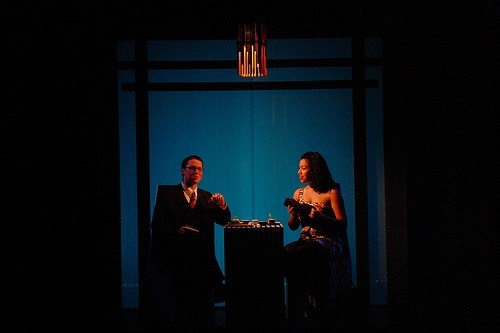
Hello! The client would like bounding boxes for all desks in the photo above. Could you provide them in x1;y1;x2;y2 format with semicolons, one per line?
223;221;285;325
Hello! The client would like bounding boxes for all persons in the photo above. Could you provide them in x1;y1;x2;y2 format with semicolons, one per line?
151;155;231;325
279;149;348;323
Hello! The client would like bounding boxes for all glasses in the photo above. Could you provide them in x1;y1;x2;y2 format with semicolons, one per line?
184;166;204;171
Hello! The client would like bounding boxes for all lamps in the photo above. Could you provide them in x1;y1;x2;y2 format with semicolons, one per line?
235;13;268;78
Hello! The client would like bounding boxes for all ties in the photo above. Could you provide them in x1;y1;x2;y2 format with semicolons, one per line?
189;191;197;209
